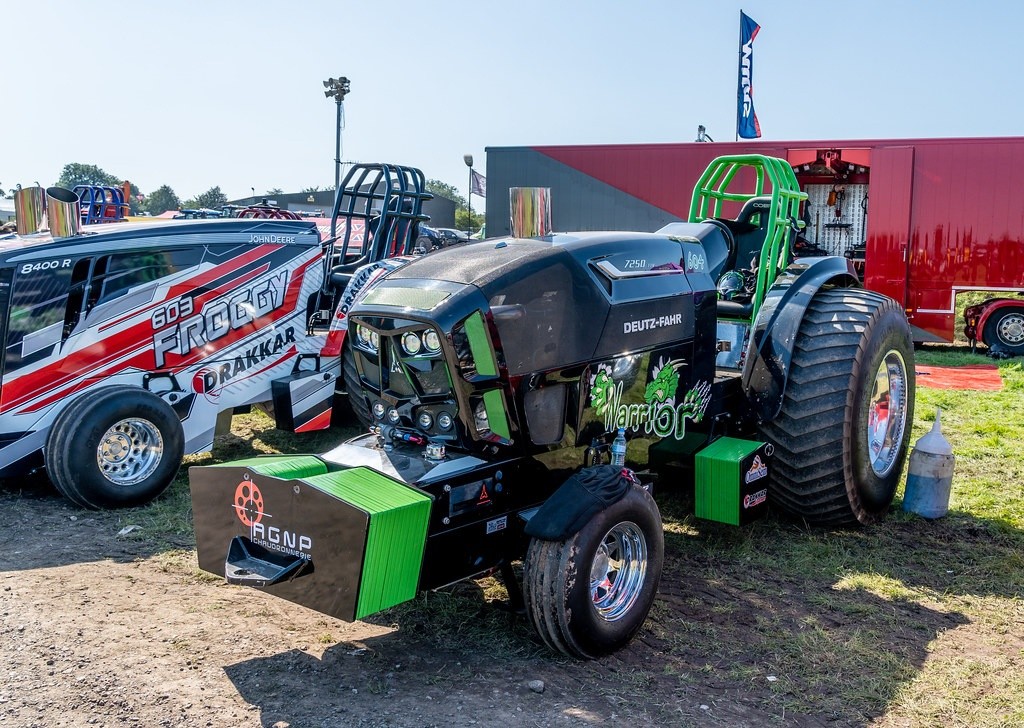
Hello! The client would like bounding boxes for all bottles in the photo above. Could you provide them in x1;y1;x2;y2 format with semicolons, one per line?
903;409;955;518
610;428;627;467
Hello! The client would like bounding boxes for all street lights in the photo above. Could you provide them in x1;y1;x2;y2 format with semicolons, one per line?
324;75;351;217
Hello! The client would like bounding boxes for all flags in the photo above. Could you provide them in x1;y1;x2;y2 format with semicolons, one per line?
471;168;486;197
738;9;761;139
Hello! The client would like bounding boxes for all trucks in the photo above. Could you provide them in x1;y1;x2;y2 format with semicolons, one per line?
484;135;1024;360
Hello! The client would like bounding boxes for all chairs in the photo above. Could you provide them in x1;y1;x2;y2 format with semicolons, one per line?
732;196;781;272
332;198;414;287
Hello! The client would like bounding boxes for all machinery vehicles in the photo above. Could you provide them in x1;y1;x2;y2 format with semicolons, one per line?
0;162;458;512
189;154;915;665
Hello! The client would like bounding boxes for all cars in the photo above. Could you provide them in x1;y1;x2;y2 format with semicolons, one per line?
435;228;469;243
